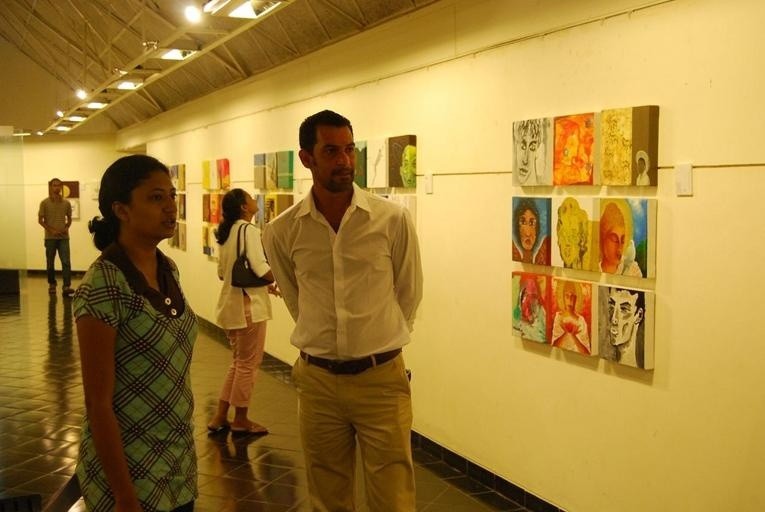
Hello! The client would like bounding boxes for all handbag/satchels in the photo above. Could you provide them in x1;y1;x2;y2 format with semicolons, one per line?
231;254;275;288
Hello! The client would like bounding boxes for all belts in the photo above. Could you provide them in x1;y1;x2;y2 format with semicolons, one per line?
299;347;403;376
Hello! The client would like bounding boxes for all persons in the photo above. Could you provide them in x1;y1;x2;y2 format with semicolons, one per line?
205;185;275;436
599;199;643;279
513;197;549;265
37;176;76;296
606;287;645;369
513;118;544;185
72;151;203;512
551;280;591;356
554;196;589;269
43;293;78;425
207;427;267;511
259;107;427;512
396;145;416;187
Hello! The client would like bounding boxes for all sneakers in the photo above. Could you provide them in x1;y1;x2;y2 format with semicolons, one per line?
49;280;57;294
62;286;75;295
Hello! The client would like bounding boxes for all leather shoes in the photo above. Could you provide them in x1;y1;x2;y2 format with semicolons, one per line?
230;422;270;434
207;419;231;432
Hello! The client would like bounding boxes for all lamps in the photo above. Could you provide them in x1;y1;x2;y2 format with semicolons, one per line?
30;0;296;137
